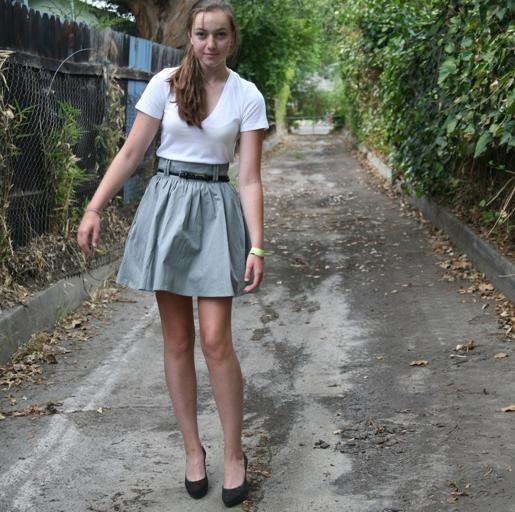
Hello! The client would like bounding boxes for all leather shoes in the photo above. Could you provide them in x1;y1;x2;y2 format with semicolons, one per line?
222;453;248;507
185;445;207;498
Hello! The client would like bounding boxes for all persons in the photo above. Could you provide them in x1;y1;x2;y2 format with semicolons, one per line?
77;0;269;507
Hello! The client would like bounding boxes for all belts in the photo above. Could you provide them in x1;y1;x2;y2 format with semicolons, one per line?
157;170;229;181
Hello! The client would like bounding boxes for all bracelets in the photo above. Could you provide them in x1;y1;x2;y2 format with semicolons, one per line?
249;247;266;258
84;208;102;216
